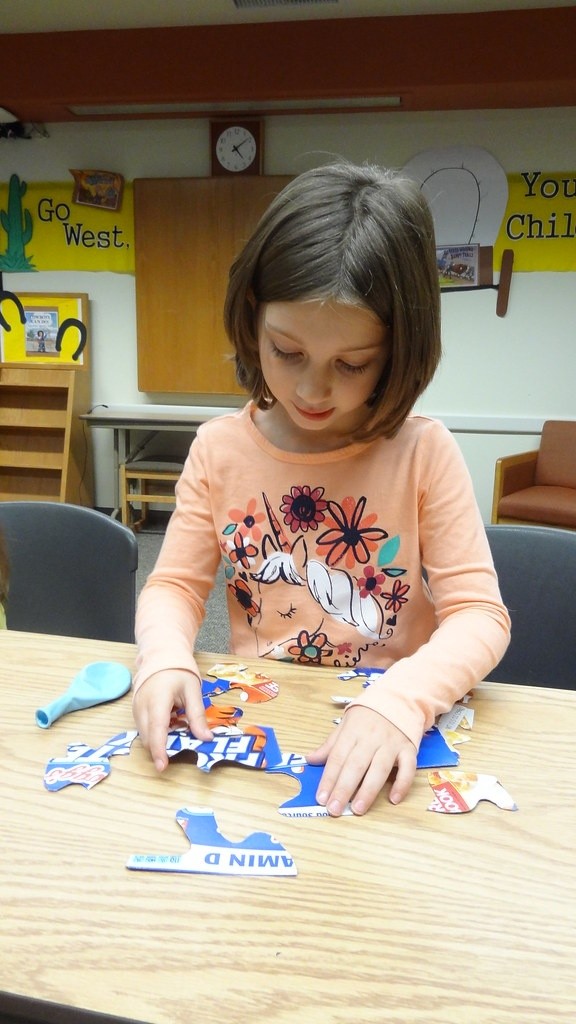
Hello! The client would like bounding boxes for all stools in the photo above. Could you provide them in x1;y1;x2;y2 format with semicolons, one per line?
120;456;189;526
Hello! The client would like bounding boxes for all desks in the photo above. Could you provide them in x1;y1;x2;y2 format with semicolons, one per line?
0;631;576;1024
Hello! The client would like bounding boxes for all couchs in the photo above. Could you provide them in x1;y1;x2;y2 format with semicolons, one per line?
491;420;576;531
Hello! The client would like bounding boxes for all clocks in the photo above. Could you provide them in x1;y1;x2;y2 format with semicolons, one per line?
209;120;264;176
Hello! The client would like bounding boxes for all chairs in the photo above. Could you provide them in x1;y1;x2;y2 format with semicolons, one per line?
483;525;576;692
0;500;139;643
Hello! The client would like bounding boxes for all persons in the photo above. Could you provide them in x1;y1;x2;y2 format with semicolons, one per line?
132;151;513;818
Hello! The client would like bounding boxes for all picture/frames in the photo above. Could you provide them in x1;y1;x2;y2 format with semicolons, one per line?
0;292;90;371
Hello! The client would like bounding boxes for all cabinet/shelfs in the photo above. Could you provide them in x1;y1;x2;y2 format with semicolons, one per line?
0;370;94;508
